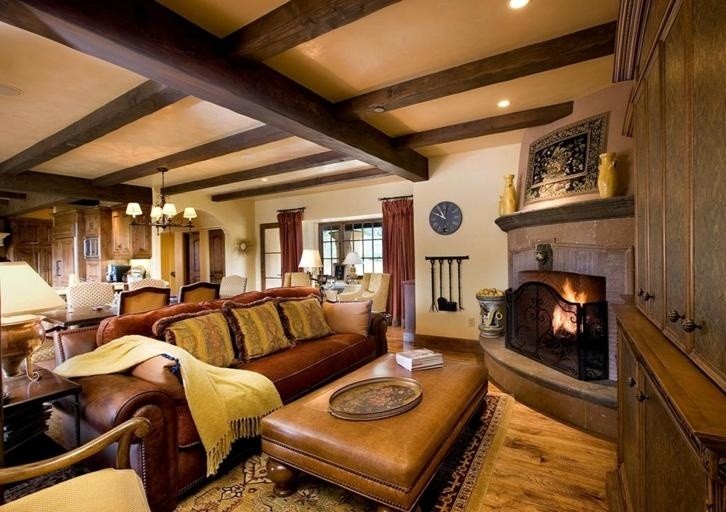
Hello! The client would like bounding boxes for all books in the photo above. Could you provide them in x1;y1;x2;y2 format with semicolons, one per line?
395;347;443;372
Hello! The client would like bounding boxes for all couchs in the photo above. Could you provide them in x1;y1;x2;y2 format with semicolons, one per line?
22;286;390;512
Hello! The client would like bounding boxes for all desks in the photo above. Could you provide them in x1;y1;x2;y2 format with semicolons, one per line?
0;363;83;469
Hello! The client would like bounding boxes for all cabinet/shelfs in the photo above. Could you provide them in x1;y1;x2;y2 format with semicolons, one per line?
111;205;135;259
52;237;81;284
628;55;663;331
615;332;708;511
660;0;726;385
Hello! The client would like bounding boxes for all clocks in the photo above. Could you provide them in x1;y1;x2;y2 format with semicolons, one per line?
429;202;463;234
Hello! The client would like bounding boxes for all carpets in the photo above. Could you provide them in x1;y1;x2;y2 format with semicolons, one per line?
157;366;513;511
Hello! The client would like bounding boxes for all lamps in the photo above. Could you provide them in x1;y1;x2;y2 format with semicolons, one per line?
297;249;323;286
125;166;198;235
342;252;363;279
0;262;67;382
